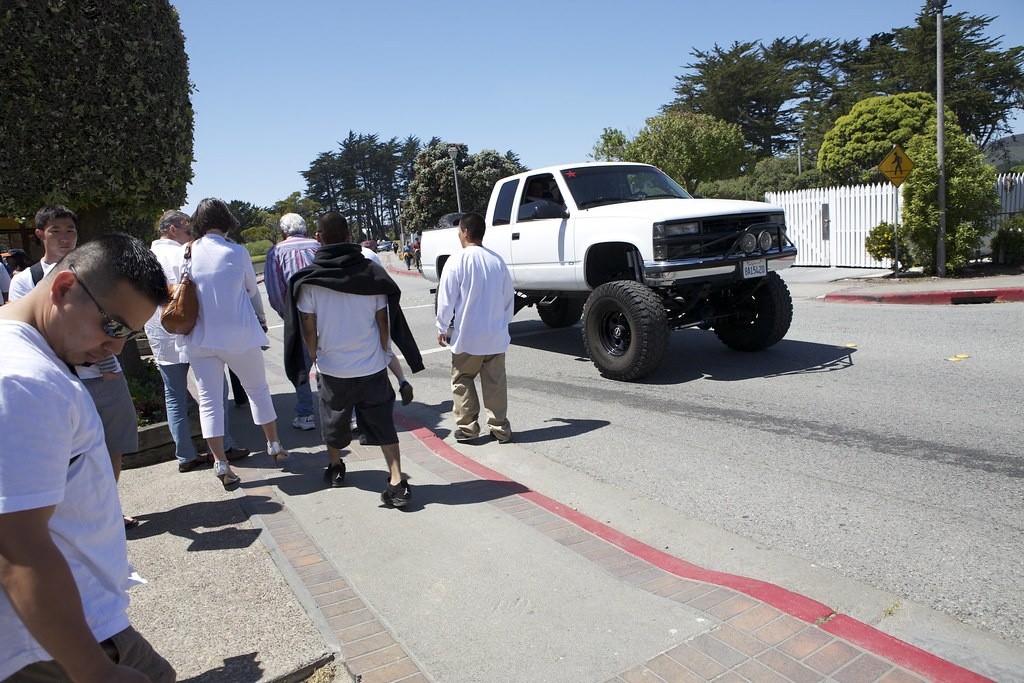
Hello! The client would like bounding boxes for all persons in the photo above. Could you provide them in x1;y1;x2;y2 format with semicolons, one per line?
435;212;514;444
413;238;420;269
0;233;170;683
264;213;322;430
144;209;251;473
284;211;414;507
393;242;398;254
8;206;139;529
402;240;413;270
177;197;290;490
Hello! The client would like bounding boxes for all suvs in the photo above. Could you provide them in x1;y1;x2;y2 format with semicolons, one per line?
360;240;377;253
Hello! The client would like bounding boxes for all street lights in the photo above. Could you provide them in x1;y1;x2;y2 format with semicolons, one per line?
448;147;461;212
395;198;406;247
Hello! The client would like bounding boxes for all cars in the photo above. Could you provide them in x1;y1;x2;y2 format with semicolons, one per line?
376;241;392;252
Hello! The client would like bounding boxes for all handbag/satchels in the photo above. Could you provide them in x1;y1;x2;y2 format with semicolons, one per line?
162;272;200;335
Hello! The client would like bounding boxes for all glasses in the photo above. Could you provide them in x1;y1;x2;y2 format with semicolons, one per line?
172;225;192;236
69;263;142;342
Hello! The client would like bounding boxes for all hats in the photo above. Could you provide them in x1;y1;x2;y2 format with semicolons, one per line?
1;249;20;258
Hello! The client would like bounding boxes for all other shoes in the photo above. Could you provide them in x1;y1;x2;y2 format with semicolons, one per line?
453;429;477;441
400;380;414;406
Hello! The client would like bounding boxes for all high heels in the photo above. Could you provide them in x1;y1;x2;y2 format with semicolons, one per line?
214;461;239;490
267;440;290;469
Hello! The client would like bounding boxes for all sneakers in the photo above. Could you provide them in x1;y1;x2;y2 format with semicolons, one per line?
178;454;207;473
350;414;359;433
380;477;412;507
324;459;346;487
208;447;251;464
292;414;316;430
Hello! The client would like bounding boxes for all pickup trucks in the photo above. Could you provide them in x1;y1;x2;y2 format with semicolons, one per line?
421;161;798;381
